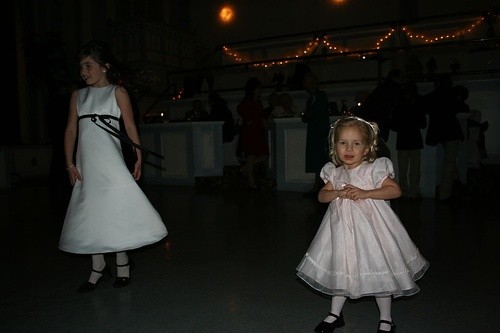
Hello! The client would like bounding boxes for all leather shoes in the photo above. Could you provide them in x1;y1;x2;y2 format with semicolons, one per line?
77;264;111;293
315;310;345;333
113;256;133;287
377;315;396;333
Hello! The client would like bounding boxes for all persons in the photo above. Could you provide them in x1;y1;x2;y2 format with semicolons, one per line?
56;42;170;294
296;116;431;333
185;70;488;203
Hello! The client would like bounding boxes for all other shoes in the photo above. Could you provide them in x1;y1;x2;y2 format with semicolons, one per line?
303;187;321;197
